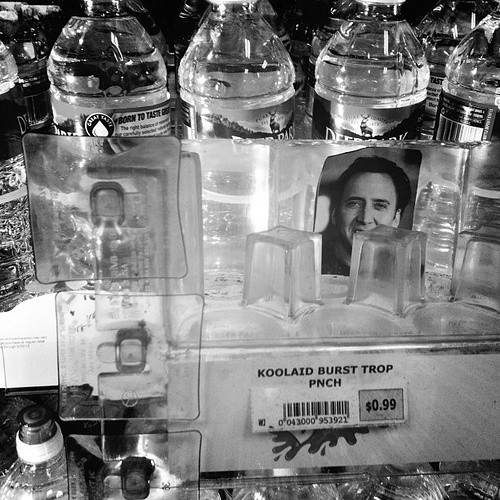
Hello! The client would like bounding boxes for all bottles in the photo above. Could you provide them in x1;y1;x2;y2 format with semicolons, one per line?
2;388;499;500
0;0;500;318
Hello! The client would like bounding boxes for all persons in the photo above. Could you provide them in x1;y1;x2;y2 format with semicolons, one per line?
321;155;414;277
